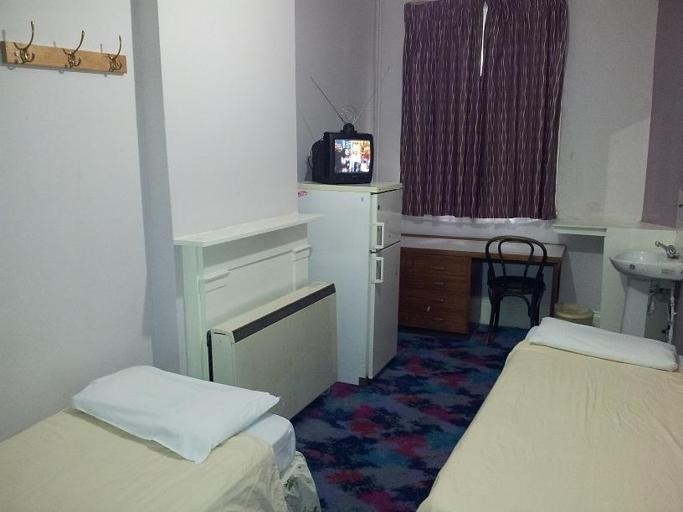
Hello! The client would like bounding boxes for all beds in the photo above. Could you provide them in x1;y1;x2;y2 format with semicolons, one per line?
0;408;320;512
417;324;683;512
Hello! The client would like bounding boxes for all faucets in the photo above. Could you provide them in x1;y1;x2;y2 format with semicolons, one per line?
655;241;680;258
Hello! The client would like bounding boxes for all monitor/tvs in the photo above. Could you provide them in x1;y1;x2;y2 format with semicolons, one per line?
311;132;374;184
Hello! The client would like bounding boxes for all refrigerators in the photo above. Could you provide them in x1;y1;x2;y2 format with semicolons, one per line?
298;181;401;386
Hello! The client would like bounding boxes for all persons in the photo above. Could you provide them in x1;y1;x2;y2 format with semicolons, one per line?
340;148;351;173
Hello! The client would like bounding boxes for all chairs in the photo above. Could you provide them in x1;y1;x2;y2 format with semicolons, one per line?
483;235;548;348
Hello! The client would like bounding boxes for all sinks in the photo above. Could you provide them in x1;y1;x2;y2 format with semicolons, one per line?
609;249;683;281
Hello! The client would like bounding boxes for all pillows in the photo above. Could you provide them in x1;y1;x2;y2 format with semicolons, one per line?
528;316;680;373
69;366;285;466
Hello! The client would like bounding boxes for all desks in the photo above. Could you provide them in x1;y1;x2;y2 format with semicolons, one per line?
399;233;567;343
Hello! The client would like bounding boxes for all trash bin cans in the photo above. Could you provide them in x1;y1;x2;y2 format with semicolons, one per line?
555;303;593;326
